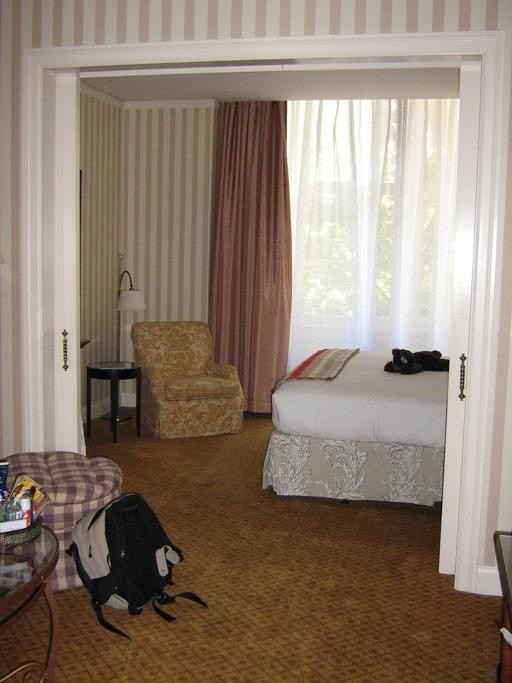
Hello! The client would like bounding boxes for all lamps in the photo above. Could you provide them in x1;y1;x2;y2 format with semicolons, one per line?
103;249;147;422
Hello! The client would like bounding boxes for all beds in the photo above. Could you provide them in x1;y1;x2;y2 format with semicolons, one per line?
261;347;449;507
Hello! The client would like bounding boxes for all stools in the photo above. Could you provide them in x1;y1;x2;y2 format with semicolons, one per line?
1;450;122;593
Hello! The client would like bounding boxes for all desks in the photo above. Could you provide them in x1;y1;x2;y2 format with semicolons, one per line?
85;360;142;445
0;523;63;683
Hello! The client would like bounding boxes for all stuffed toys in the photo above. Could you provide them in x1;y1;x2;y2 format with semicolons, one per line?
384;347;449;374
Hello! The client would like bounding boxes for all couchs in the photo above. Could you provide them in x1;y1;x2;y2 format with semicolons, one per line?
131;318;248;440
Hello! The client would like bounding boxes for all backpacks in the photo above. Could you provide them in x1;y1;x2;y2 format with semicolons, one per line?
65;493;208;641
